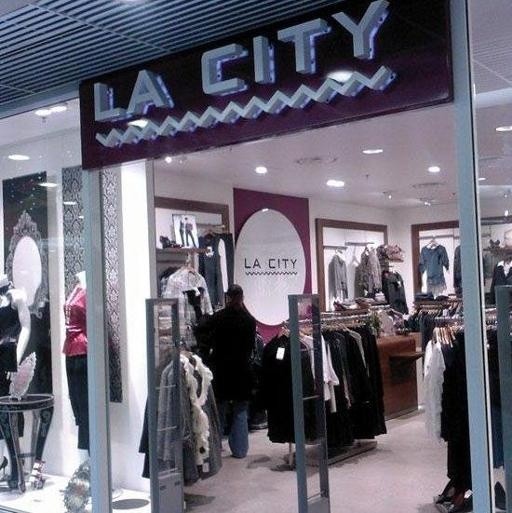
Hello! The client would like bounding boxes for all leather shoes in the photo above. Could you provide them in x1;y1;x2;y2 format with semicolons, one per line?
436;476;457;504
449;494;472;512
495;481;506;509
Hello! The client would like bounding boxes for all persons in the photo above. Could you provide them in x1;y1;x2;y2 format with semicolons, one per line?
62;270;90;457
194;284;255;458
489;245;512;305
0;273;34;483
179;215;185;247
184;218;196;248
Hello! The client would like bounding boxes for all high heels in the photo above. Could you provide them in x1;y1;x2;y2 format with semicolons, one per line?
0;455;9;477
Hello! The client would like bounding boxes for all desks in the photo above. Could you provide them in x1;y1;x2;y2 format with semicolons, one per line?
0;393;54;485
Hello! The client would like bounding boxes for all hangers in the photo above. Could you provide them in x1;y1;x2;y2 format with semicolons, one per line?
417;232;445;251
264;310;371;353
414;301;512;357
157;262;206;286
329;242;374;266
